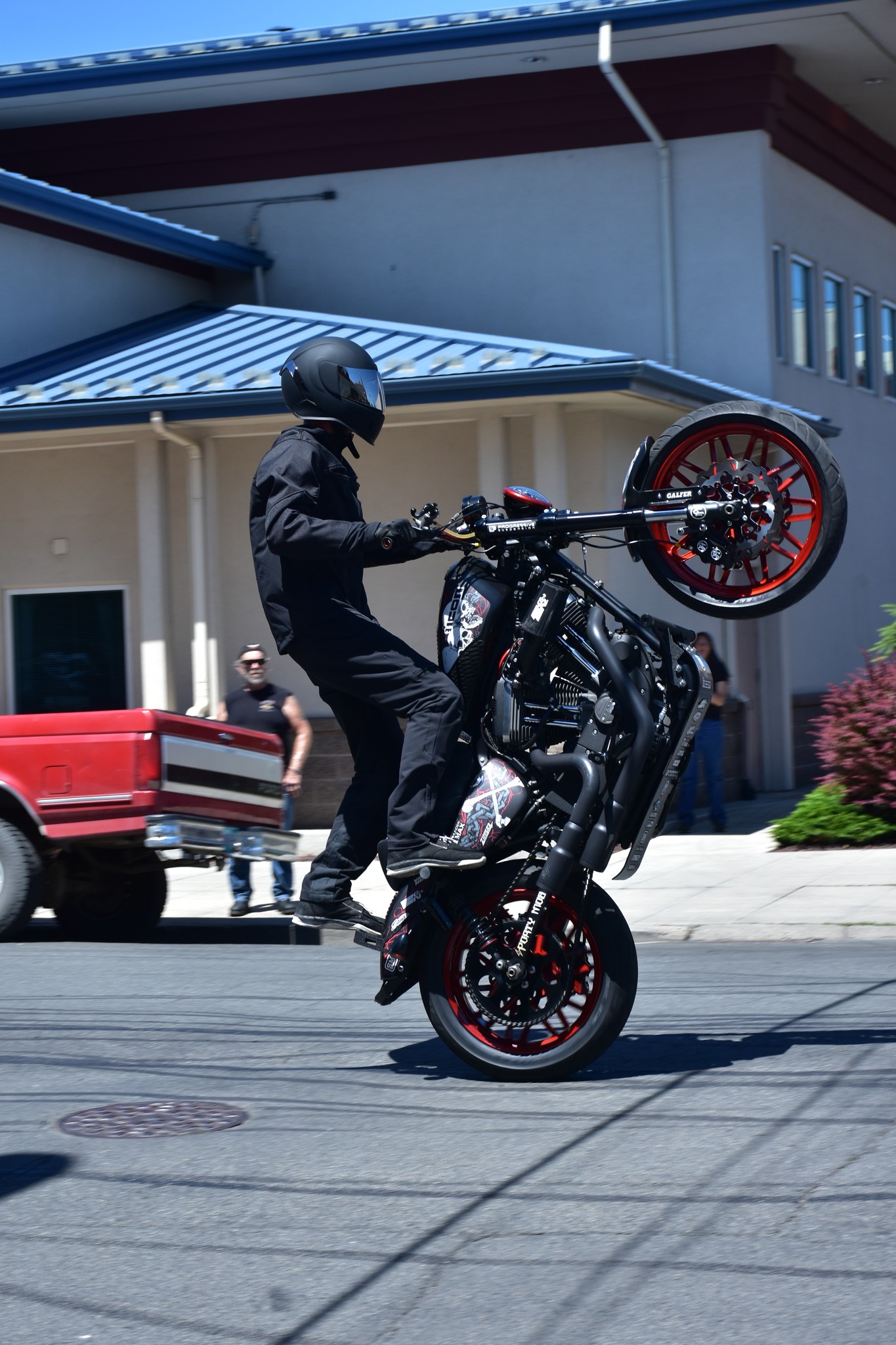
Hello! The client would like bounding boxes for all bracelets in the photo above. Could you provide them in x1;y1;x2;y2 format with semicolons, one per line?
287;767;304;776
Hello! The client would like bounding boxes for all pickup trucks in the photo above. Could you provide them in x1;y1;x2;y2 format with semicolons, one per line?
0;708;299;939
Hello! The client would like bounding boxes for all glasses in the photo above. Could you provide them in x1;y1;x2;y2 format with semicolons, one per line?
242;658;265;666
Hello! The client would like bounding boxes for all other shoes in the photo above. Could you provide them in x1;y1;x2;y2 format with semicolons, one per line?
277;898;297;915
679;825;693;833
231;901;248;916
714;822;728;832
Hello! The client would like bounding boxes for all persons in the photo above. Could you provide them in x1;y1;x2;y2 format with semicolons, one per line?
672;631;728;835
251;343;487;928
214;643;314;917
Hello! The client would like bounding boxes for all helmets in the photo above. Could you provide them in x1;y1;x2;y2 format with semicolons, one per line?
278;337;384;446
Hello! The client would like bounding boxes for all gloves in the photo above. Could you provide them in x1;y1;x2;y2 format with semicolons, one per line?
374;518;417;547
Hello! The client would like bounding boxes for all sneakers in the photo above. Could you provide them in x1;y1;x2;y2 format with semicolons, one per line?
291;899;384;937
385;838;486;878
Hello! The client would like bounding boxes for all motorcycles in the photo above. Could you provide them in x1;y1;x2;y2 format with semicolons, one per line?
370;399;850;1078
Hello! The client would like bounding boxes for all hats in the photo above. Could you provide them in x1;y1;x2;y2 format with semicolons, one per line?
238;643;266;659
